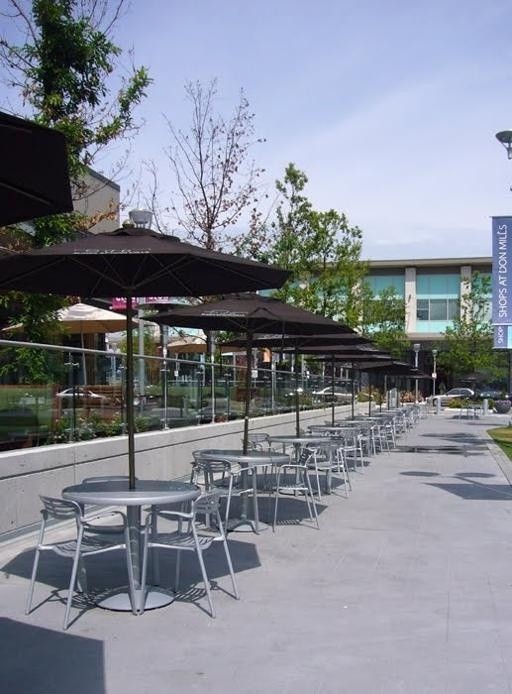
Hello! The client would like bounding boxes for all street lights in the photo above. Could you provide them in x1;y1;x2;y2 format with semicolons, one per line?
127;207;157;396
494;129;512;191
431;348;438;397
413;342;420;406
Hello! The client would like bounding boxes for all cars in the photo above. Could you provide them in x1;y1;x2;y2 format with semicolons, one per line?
51;385;111;405
138;405;200;420
282;386;311;398
473;387;512;406
181;388;281;421
123;393;161;412
426;387;476;405
311;385;358;402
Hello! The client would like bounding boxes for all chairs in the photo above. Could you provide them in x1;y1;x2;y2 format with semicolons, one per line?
457;399;490;422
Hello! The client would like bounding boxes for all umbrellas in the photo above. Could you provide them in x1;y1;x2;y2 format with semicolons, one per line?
0;294;138;417
0;217;296;490
0;109;75;228
